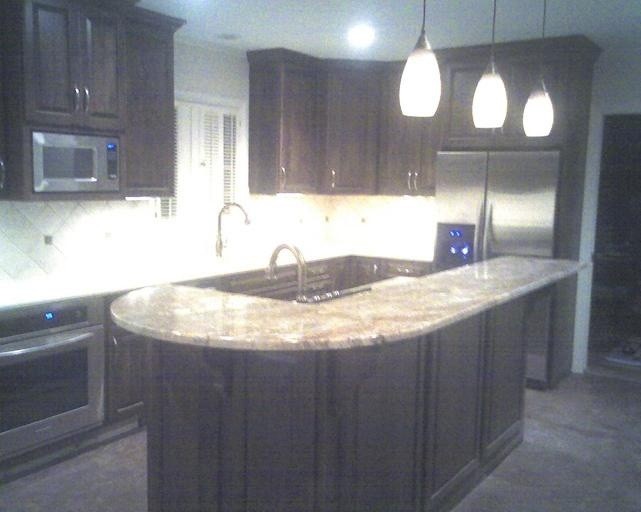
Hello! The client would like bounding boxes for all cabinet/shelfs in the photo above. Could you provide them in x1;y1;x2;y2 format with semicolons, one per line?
352;255;435;288
322;62;379;195
250;52;320;193
0;2;123;128
379;62;443;196
228;258;350;298
445;33;604;148
105;278;221;422
120;7;176;198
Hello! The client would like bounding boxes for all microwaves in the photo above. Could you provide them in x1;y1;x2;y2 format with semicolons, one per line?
30;128;120;194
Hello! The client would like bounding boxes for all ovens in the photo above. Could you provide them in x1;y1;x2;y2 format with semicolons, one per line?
0;294;106;460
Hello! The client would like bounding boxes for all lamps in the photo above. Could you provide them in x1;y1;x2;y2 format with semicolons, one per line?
398;2;444;119
522;0;556;139
471;0;509;129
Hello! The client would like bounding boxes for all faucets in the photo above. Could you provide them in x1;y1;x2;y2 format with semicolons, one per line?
216;198;252;264
264;241;312;303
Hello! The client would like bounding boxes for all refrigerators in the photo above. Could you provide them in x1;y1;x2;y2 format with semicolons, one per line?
433;149;559;388
